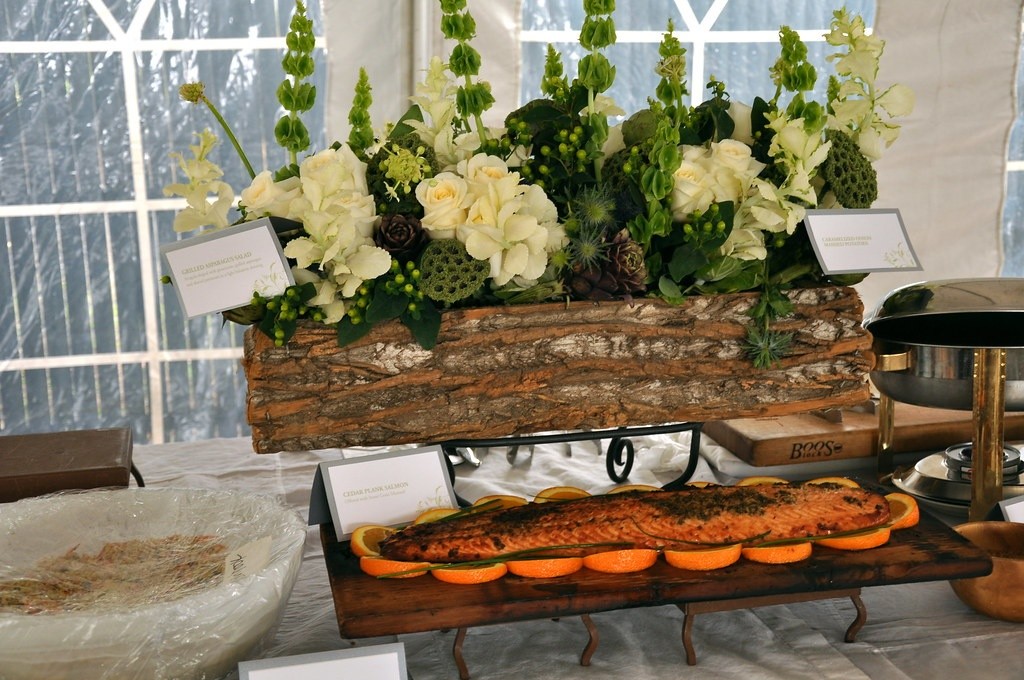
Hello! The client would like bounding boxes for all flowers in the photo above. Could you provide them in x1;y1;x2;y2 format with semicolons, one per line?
165;0;915;348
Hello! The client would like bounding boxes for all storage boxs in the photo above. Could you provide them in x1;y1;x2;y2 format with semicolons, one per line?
697;401;1022;465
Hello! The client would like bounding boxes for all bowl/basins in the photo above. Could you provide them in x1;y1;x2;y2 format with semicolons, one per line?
947;521;1024;624
0;489;308;680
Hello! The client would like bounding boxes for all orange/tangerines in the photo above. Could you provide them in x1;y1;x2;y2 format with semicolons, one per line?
350;475;918;583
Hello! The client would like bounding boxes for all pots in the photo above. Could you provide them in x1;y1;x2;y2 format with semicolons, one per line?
890;441;1024;518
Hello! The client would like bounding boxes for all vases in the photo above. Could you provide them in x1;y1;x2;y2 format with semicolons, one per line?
245;270;880;452
